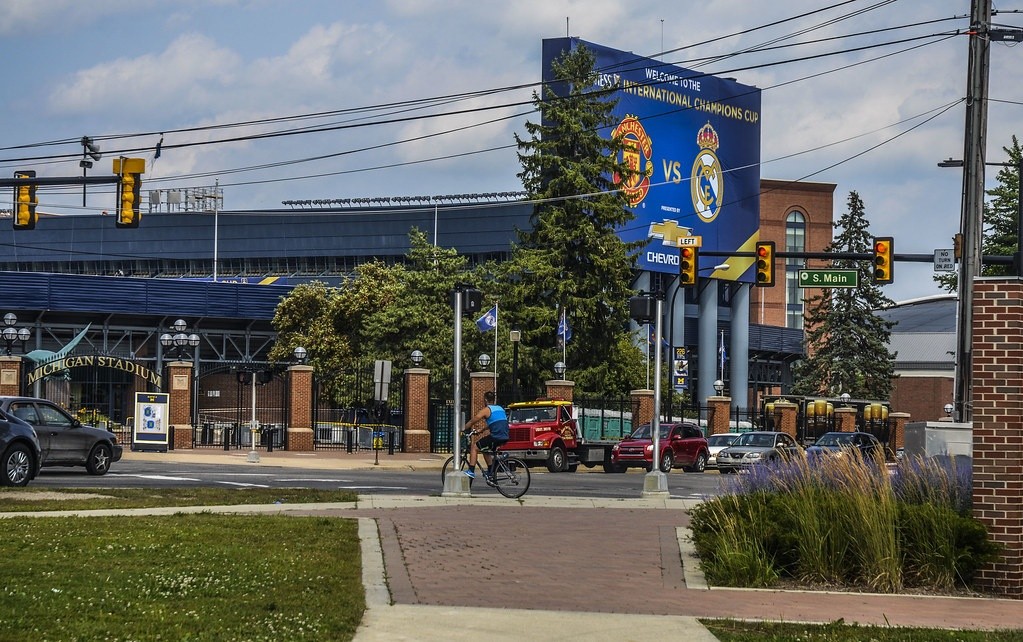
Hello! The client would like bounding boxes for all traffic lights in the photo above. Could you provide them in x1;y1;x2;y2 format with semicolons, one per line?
258;370;274;383
875;240;890;281
757;245;772;284
236;370;252;384
680;247;694;284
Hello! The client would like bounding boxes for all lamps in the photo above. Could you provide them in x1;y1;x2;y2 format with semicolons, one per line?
554;362;566;380
158;319;200;361
478;353;491;372
282;189;532;208
712;379;724;396
943;403;954;417
293;346;307;365
839;393;851;408
410;350;424;368
0;312;32;355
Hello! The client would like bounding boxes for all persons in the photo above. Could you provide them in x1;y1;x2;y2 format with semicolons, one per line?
463;392;510;479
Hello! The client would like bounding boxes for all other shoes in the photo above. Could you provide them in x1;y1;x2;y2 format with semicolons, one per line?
463;469;475;479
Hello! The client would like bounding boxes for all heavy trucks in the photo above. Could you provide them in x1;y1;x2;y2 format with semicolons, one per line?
498;399;634;476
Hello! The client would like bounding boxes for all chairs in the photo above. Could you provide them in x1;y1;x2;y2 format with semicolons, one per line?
15;408;37;425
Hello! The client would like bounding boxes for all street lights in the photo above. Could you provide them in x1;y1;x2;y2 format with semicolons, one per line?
668;264;729;423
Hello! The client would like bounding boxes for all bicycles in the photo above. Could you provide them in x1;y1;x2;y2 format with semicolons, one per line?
442;429;530;498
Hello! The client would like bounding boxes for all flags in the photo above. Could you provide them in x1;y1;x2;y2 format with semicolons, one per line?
475;306;496;332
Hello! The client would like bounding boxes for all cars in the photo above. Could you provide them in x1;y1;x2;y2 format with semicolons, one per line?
803;431;883;465
612;421;710;472
716;431;800;475
703;433;744;470
0;395;122;476
0;407;40;487
